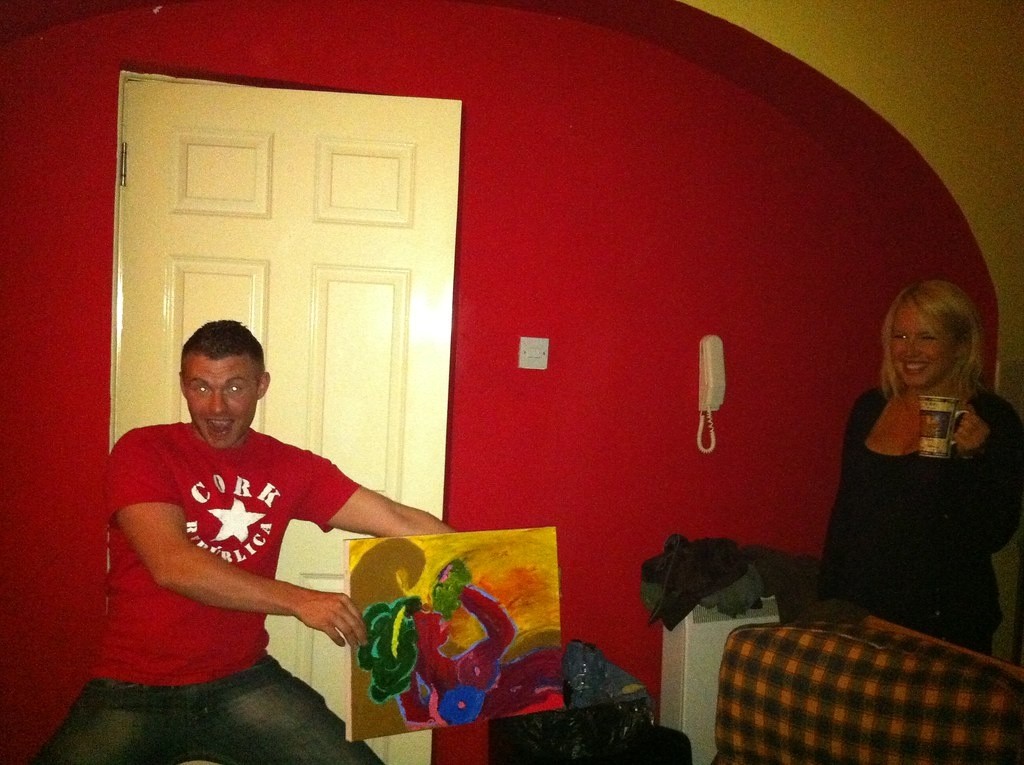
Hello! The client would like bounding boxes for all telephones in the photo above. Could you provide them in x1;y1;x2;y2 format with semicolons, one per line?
698;334;726;412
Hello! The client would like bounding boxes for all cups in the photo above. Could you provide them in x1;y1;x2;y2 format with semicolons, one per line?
917;395;969;459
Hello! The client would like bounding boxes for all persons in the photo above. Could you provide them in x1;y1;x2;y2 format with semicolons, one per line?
32;320;458;765
819;278;1024;656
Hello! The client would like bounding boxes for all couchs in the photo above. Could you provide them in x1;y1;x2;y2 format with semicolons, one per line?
709;607;1024;765
